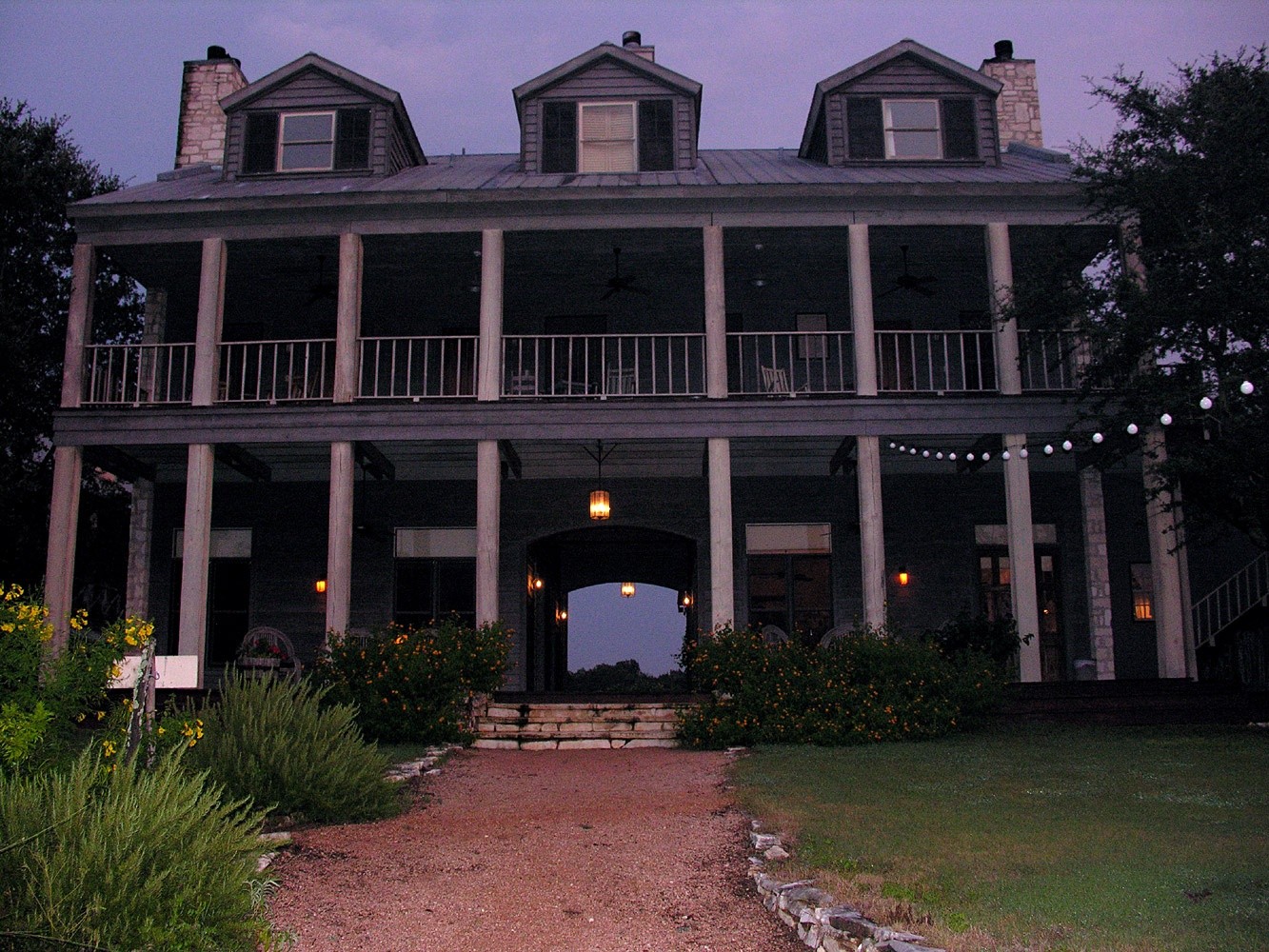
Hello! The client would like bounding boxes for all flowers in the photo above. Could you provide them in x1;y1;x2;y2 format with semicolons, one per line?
238;640;282;658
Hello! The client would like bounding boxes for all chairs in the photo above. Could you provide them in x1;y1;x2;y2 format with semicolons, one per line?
820;622;863;651
760;365;811;400
762;625;788;645
285;365;320;398
244;625;302;682
604;363;640;400
318;631;373;652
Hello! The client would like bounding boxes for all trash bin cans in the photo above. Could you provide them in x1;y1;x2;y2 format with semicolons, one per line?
1073;656;1097;681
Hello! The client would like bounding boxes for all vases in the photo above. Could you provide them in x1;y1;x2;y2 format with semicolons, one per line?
240;658;280;689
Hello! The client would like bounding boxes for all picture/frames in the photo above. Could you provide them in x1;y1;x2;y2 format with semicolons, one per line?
795;312;829;361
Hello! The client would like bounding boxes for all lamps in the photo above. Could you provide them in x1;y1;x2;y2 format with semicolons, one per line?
620;581;637;600
585;441;617;521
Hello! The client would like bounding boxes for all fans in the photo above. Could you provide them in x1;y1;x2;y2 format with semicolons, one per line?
284;254;338;300
581;248;653;302
875;245;938;298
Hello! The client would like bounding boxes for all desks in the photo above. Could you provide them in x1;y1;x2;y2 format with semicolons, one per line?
510;369;541;398
188;381;229;404
548;381;597;398
